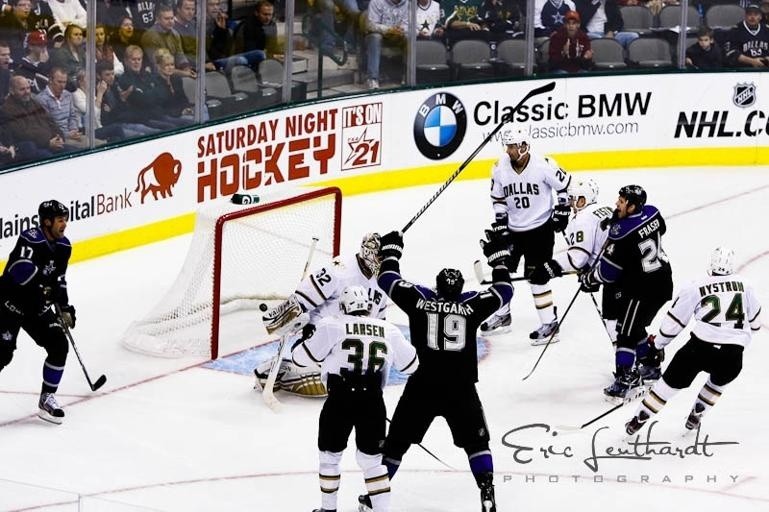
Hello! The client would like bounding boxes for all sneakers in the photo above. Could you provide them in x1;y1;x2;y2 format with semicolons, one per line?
480;313;511;331
603;372;642;398
364;78;379;90
38;392;65;417
358;494;372;509
481;486;496;512
684;409;702;430
528;320;560;339
624;415;647;435
633;366;662;380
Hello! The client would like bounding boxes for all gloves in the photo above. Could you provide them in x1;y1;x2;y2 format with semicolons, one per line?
528;260;563;285
645;343;665;367
380;230;405;260
479;229;514;268
577;268;601;293
61;305;76;329
302;323;317;340
553;204;571;233
491;213;508;232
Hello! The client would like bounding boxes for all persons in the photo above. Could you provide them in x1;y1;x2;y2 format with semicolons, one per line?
2;1;767;167
527;175;621;353
577;182;673;396
619;246;767;435
255;233;388;399
0;199;78;416
358;226;514;512
290;285;420;512
479;131;577;341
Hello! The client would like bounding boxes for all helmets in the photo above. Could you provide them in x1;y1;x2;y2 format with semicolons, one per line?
706;243;737;276
338;284;369;314
566;176;600;210
435;268;465;295
618;185;647;207
358;232;383;279
38;199;69;223
502;125;530;146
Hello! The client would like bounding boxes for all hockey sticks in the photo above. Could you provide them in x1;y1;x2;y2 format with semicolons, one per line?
522;237;608;381
262;236;320;414
473;260;578;285
387;418;469;472
555;387;652;431
54;300;107;391
401;82;556;234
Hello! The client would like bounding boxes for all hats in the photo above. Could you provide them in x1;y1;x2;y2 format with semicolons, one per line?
564;11;581;21
26;29;48;46
745;2;761;12
